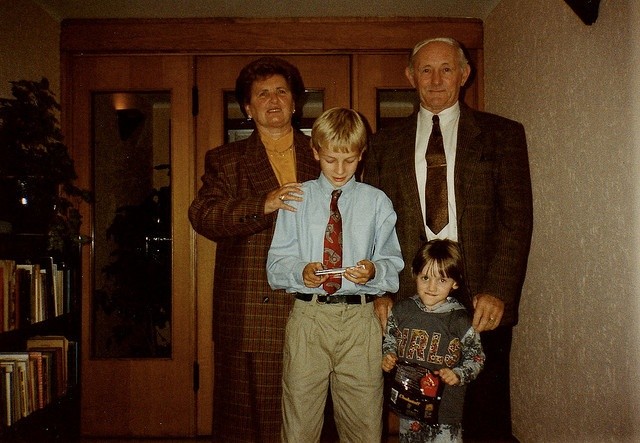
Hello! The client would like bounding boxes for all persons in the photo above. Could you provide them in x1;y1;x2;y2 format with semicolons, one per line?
361;36;535;443
380;238;487;443
265;106;406;443
187;53;322;442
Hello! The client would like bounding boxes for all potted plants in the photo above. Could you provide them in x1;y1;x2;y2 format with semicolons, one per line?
0;75;98;261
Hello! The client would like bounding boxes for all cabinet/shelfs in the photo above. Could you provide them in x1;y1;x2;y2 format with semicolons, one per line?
351;48;481;177
0;260;81;434
63;49;195;439
196;52;351;438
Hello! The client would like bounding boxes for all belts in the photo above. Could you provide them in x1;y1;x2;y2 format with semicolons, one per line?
296;293;373;305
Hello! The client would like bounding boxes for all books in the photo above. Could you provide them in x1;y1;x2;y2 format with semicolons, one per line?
0;335;78;427
0;255;72;333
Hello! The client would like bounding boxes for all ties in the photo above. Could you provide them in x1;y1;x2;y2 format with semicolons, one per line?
322;190;343;294
425;115;450;235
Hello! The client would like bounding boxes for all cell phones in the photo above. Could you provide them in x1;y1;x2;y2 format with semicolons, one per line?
315;265;366;278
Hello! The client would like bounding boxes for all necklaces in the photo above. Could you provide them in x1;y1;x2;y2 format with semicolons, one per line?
264;143;295;157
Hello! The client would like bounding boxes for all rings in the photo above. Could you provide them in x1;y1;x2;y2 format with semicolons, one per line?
278;193;287;200
488;317;495;325
